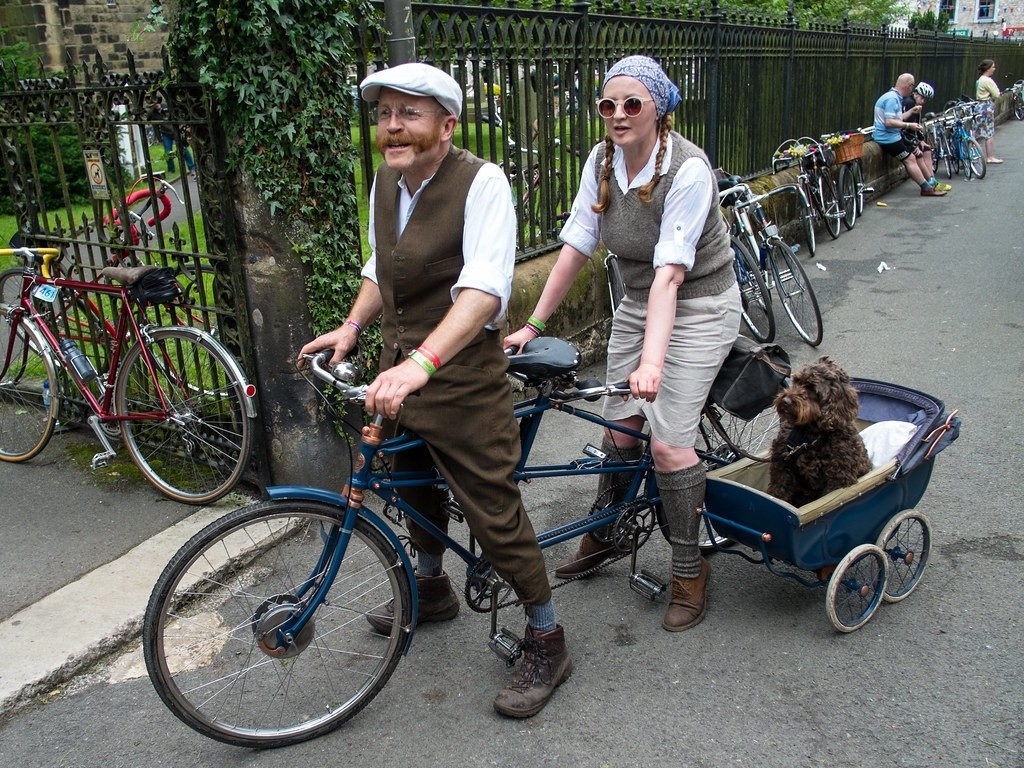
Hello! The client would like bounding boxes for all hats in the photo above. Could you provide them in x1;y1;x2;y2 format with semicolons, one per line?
360;63;463;125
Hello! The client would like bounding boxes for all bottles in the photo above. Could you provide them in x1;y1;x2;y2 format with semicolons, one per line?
59;336;96;382
42;381;60;425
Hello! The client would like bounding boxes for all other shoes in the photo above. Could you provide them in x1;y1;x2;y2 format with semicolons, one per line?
988;156;1004;164
921;187;947;196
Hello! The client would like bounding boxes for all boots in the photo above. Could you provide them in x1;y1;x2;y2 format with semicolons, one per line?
367;569;460;635
495;619;569;718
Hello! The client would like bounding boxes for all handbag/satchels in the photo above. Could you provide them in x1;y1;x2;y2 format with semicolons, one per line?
709;334;792;424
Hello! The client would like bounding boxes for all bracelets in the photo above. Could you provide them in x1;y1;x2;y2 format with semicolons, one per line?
408;349;437;376
525;316;546;336
905;124;910;129
418;346;441;369
345;320;361;332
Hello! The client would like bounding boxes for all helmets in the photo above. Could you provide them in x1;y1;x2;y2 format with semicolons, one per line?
915;81;934;100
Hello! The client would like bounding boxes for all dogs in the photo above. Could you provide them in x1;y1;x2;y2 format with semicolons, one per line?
766;355;872;503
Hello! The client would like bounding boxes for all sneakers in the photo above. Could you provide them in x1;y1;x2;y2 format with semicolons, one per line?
663;557;710;632
555;533;629;577
935;182;952;191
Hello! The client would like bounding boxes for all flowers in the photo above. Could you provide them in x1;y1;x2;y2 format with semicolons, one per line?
819;130;855;149
772;141;817;161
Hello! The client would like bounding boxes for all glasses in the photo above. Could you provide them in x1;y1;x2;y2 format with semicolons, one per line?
369;105;446;123
595;97;654;118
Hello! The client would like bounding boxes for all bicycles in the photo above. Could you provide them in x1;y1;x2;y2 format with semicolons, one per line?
0;177;238;397
716;176;825;348
1009;79;1024;121
772;134;875;257
921;96;987;182
0;247;262;507
141;320;966;749
482;103;591;250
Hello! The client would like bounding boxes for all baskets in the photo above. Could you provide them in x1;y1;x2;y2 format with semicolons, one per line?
832;134;865;164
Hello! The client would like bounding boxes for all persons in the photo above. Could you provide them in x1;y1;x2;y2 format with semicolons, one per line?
973;59;1004;163
574;71;598;99
553;71;559;118
296;64;572;720
503;54;743;631
149;103;195;176
901;82;952;191
872;73;947;196
